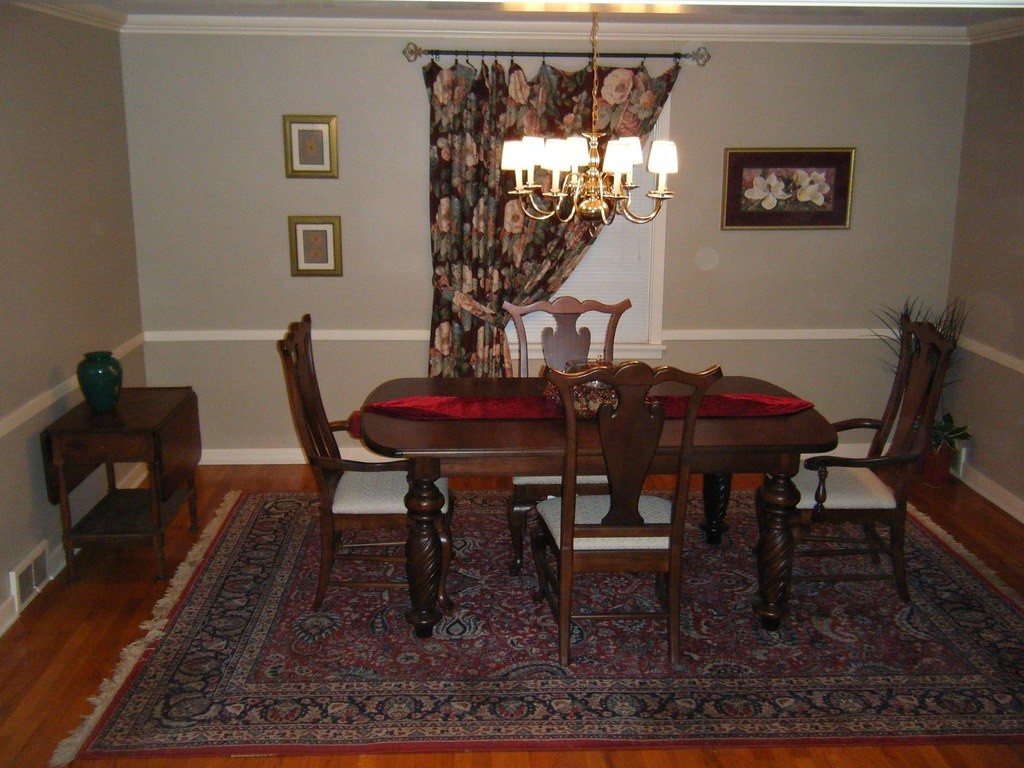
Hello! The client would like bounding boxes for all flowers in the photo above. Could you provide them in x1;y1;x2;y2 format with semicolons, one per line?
544;355;620;415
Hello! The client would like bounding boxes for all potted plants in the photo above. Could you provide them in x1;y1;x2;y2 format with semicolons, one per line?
866;296;972;488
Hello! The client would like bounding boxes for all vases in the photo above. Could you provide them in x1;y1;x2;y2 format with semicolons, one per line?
77;350;121;413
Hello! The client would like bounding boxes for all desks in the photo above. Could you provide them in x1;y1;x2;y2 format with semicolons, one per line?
39;385;203;583
361;376;839;637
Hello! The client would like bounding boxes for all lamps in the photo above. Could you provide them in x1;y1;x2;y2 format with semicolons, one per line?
500;12;678;237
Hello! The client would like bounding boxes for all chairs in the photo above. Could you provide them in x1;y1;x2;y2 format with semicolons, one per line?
750;312;958;601
502;297;633;577
279;314;456;614
530;362;724;665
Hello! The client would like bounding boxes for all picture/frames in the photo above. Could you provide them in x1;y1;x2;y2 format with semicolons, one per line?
721;147;855;230
289;216;342;277
283;114;339;179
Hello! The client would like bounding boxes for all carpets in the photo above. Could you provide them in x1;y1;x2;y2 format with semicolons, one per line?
48;489;1024;765
